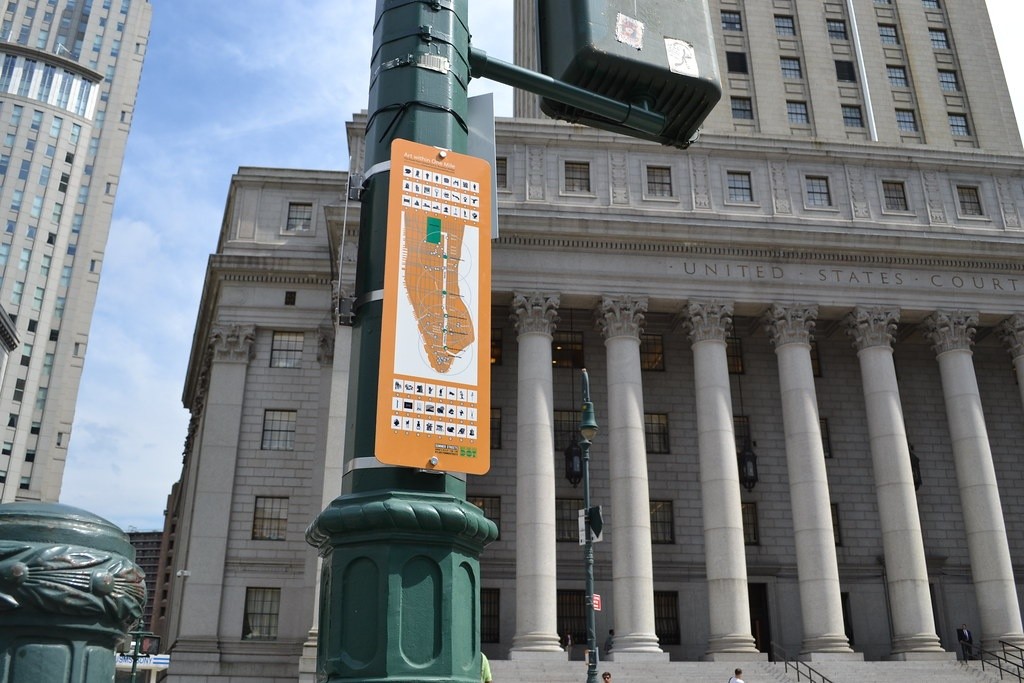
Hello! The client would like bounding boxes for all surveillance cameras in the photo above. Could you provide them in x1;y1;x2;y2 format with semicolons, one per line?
177;571;182;577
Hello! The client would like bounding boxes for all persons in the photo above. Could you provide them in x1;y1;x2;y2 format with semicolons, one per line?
958;624;973;661
480;652;492;683
604;629;615;655
602;672;612;683
728;668;745;683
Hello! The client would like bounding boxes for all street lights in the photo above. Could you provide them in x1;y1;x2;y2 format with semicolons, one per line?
568;367;604;683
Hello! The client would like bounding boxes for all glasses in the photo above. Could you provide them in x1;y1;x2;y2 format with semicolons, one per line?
604;677;610;680
